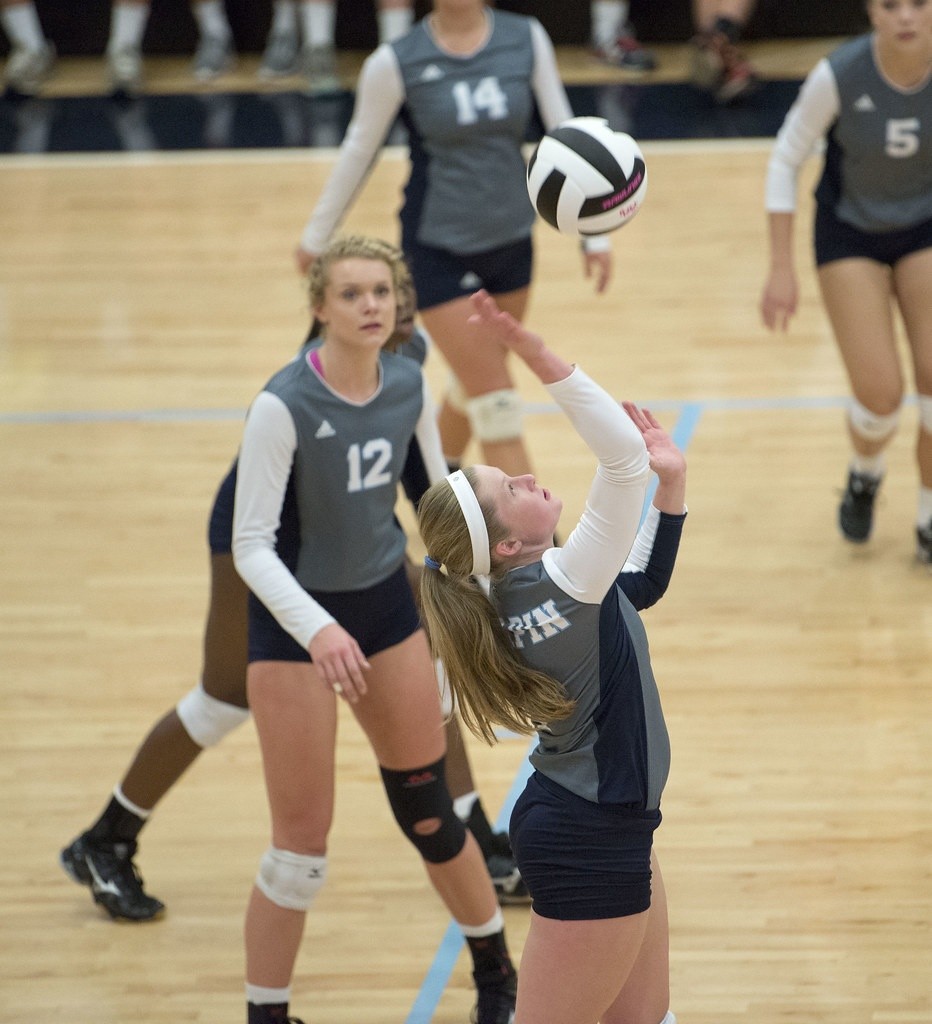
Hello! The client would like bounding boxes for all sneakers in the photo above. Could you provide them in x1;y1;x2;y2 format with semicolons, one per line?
840;469;883;543
61;835;167;924
916;523;932;564
248;1002;304;1024
480;835;535;905
471;970;518;1024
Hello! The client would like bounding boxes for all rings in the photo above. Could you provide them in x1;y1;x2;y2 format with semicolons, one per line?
332;682;344;696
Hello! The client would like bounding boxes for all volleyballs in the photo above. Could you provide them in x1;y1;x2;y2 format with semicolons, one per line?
523;115;649;240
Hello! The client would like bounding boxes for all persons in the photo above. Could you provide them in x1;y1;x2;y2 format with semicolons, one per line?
293;0;613;549
232;238;519;1024
58;235;532;925
761;0;932;564
0;0;757;108
416;287;691;1024
